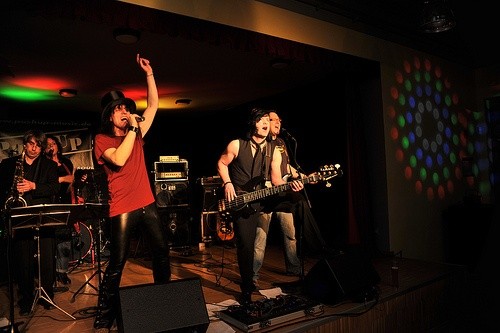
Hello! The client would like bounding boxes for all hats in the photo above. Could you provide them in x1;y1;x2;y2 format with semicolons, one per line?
100;91;137;126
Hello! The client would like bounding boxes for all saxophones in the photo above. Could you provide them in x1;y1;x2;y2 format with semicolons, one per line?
5;148;28;207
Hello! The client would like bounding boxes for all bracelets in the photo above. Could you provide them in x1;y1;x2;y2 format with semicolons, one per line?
128;126;138;133
146;74;153;77
224;181;231;186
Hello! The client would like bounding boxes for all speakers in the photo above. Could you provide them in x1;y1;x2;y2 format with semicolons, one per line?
154;181;188;207
302;245;383;300
116;277;210;333
157;204;190;247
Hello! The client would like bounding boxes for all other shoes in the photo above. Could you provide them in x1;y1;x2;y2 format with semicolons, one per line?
289;270;307;276
243;292;268;302
19;296;35;316
37;295;57;310
254;280;259;286
303;262;316;274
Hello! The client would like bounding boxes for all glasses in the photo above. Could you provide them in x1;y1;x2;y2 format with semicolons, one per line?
270;119;282;122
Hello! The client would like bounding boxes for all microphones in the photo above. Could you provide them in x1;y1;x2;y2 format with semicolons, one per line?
135;116;145;122
49;147;54;158
281;128;296;140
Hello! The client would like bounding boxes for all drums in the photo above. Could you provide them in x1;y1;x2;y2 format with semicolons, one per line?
65;221;93;263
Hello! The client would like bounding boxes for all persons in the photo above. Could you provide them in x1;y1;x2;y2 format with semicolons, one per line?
252;109;318;294
93;55;171;333
0;130;87;316
217;109;303;311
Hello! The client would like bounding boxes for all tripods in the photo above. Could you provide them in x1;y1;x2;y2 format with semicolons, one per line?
10;206;78;329
68;205;110;303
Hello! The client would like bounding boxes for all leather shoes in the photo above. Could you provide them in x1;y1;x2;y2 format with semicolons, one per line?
57;272;71;284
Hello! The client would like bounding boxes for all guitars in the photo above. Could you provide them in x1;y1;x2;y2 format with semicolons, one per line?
216;164;343;223
